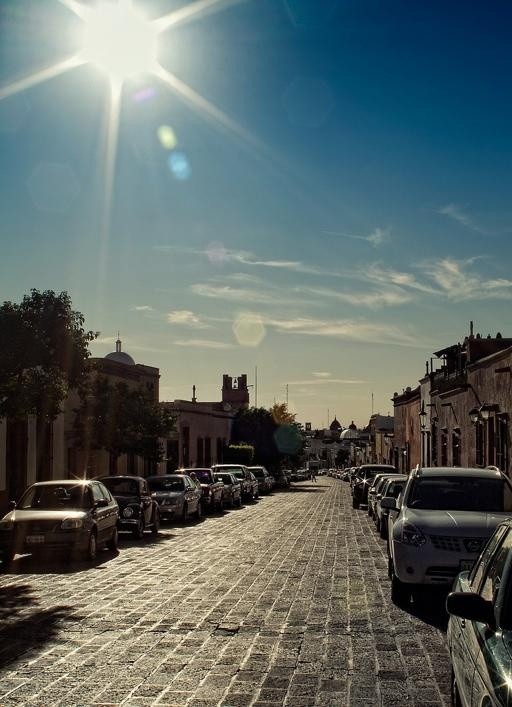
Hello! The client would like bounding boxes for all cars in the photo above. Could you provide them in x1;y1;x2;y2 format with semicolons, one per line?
174;464;276;513
144;474;202;523
444;521;511;707
349;463;512;603
1;479;119;560
96;475;160;539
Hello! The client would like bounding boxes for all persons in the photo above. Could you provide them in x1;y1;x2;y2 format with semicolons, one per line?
312;469;316;482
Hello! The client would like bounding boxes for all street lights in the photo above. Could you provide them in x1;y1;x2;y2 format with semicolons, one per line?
418;399;428;466
384;431;390;464
468;401;508;476
